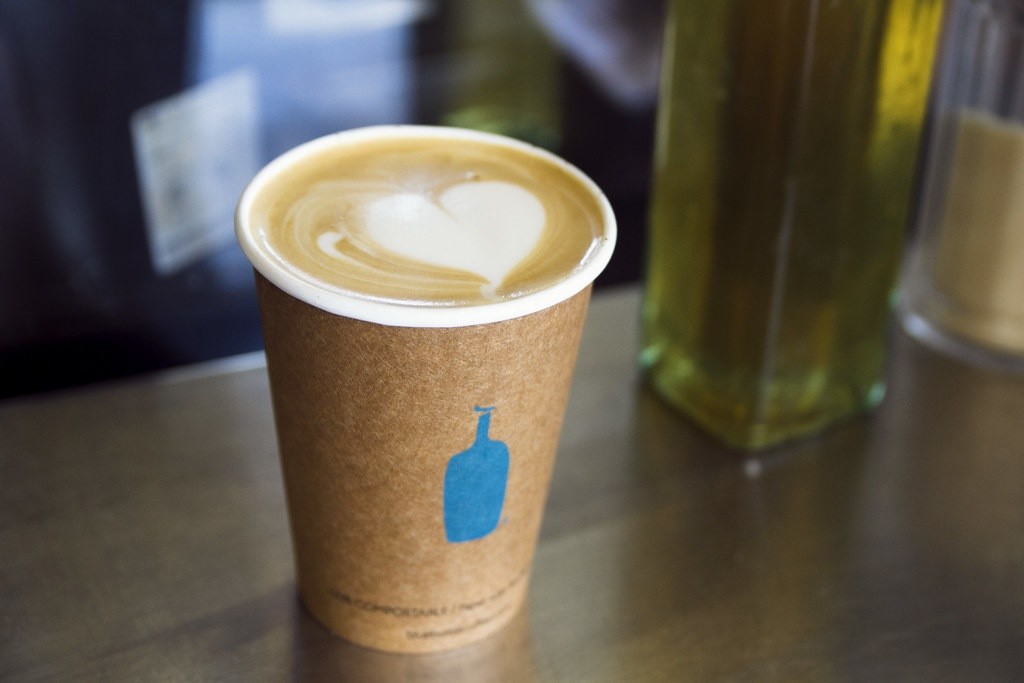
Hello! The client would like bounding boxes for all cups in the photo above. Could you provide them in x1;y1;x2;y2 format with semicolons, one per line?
235;121;620;655
888;0;1024;377
640;0;945;448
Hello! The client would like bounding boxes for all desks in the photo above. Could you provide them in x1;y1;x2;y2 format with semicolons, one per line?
0;247;1024;683
